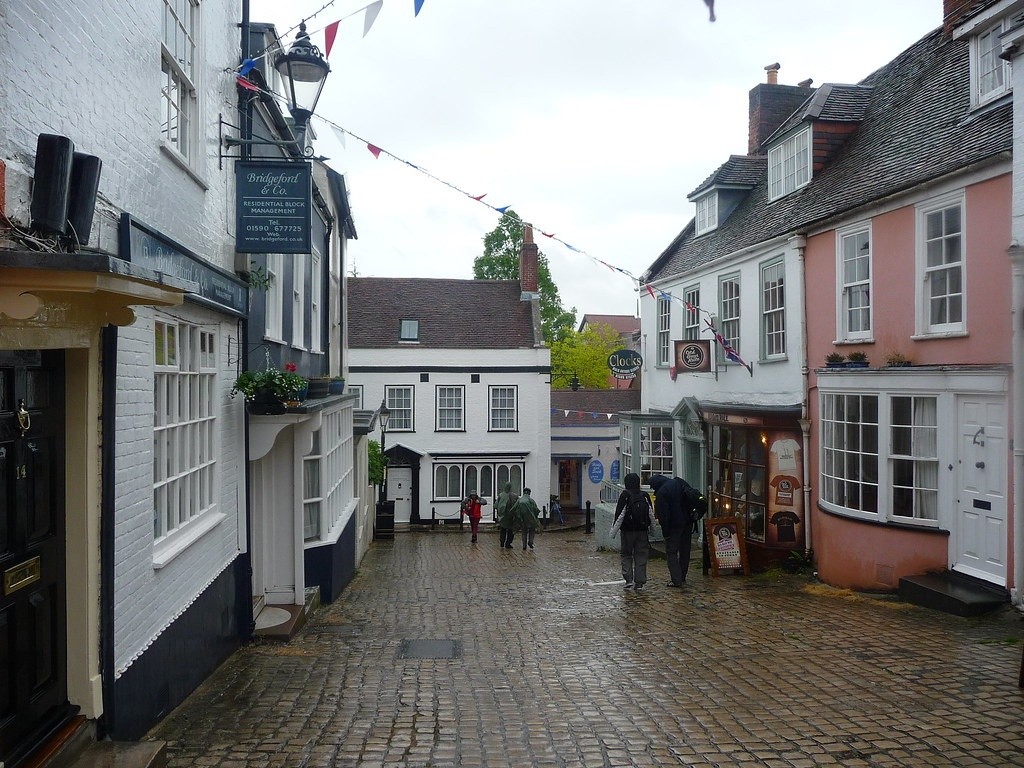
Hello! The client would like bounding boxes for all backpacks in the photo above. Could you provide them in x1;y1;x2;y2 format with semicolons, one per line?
676;477;707;523
624;488;650;531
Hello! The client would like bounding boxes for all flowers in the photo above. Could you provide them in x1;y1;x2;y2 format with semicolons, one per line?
230;362;310;400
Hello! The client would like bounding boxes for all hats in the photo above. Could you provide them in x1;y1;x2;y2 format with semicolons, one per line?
470;490;476;495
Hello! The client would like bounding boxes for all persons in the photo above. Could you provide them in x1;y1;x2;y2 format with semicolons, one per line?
649;474;694;588
612;473;653;590
509;488;539;549
461;490;487;543
494;482;518;548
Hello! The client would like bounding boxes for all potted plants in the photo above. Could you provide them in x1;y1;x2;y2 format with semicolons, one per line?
847;351;870;368
330;376;346;394
823;352;846;368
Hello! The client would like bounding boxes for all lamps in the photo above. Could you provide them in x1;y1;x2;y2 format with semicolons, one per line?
551;369;584;391
31;133;74;249
58;151;103;250
225;20;332;150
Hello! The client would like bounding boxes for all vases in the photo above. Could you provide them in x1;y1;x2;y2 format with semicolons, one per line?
245;396;287;415
887;361;912;367
290;382;309;402
305;376;330;398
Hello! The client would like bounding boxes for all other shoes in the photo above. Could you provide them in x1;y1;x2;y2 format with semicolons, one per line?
528;543;534;548
667;580;683;587
623;580;633;588
472;539;478;543
523;546;526;551
635;582;643;589
505;545;514;549
501;543;504;547
